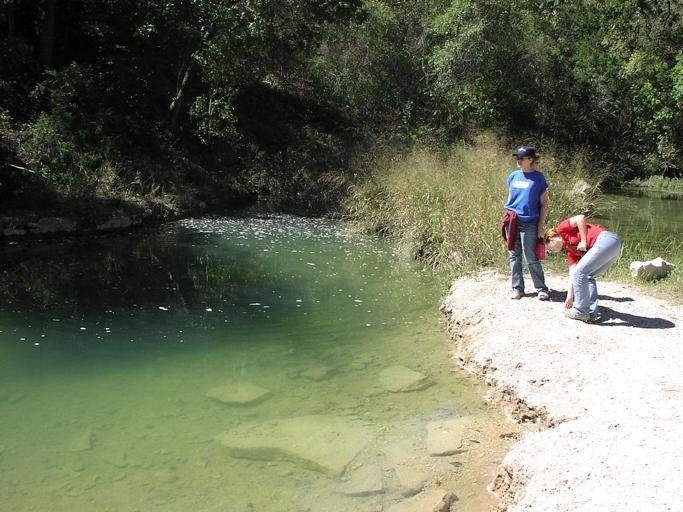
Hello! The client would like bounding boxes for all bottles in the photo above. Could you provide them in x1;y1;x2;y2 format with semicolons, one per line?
535;235;546;261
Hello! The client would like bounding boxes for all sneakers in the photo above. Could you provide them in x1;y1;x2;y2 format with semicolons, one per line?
511;289;524;300
537;290;550;301
564;310;597;322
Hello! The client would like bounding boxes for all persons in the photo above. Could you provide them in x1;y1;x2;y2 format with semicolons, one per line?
504;147;549;302
543;214;622;322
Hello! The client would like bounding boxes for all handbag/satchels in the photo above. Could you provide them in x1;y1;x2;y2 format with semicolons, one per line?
534;237;545;261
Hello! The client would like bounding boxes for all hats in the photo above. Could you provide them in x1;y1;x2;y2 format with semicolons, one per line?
511;146;537;158
